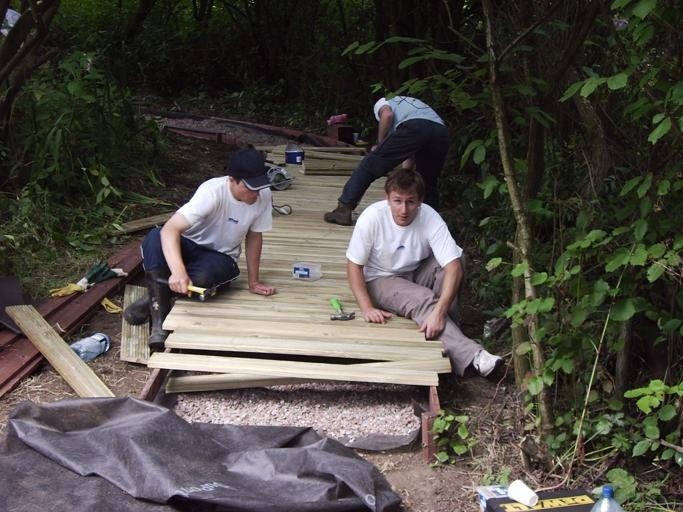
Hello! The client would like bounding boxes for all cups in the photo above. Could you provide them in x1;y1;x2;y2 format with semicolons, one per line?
506;478;538;506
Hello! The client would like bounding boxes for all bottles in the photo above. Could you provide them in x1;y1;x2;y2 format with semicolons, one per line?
283;137;304;178
70;333;112;363
590;485;624;511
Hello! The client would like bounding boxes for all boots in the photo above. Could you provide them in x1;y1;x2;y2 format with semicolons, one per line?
125;267;172;347
324;200;354;226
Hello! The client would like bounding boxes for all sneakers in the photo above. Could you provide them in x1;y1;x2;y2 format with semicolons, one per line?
471;349;503;378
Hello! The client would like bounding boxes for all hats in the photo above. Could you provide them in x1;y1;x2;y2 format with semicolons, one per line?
229;148;272;191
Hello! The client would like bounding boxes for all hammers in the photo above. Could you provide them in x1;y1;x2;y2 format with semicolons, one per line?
264;159;286;167
330;298;355;321
155;278;219;302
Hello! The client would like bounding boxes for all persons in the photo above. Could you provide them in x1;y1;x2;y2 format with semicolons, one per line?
324;95;451;226
124;151;276;351
346;168;504;378
